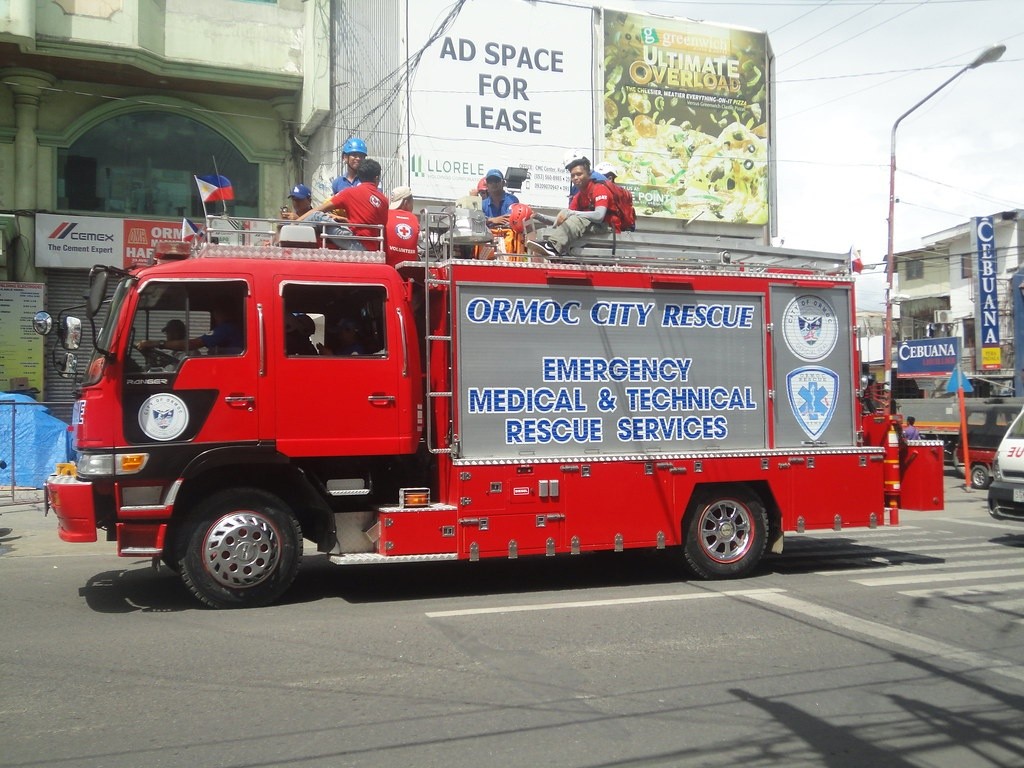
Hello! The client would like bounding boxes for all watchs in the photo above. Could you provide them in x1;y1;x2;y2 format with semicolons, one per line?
159;340;166;349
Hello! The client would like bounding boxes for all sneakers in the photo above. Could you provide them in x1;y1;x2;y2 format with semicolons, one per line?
527;239;558;260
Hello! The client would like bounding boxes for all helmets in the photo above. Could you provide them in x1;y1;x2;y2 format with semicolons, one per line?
594;163;618;177
477;177;488;192
510;204;532;233
343;138;367;156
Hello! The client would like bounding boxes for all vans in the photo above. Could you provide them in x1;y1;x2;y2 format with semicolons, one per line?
988;406;1024;521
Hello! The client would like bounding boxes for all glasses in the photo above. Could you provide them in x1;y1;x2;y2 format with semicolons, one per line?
486;178;500;184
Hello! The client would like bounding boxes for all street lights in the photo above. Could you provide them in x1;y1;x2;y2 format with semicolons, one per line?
883;44;1008;414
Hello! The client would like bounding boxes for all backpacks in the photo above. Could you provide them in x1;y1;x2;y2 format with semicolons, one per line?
578;179;637;232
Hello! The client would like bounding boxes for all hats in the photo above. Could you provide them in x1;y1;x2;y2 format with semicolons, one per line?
486;169;503;180
357;160;381;180
388;186;411;209
162;320;185;332
288;184;311;198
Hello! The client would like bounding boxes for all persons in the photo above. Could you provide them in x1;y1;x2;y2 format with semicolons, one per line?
292;159;389;252
161;319;201;357
902;417;922;440
284;313;385;357
383;186;421;270
470;156;619;262
283;184;313;220
136;297;246;356
332;137;383;198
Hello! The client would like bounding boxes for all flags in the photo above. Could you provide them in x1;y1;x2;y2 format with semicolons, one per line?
848;245;863;274
195;173;235;203
182;217;207;242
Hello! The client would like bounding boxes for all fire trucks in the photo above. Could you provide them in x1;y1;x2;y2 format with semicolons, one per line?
32;209;886;609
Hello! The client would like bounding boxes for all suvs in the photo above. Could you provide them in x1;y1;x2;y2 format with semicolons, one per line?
954;406;1022;489
